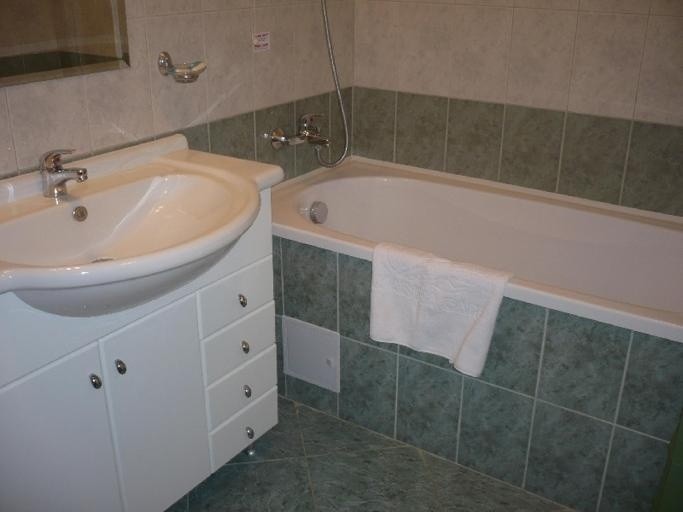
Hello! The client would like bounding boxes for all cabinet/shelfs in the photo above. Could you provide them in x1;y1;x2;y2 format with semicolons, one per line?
0;291;209;511
193;252;277;476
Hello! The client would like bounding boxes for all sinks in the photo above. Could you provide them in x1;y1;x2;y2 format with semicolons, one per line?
0;173;262;314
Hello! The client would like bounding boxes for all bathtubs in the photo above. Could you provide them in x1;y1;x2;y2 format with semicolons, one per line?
270;155;683;342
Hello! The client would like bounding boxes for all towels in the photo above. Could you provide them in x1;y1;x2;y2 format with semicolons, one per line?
368;242;512;375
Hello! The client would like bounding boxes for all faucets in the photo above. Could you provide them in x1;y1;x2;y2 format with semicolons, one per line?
39;148;86;197
300;113;333;150
175;59;206;76
